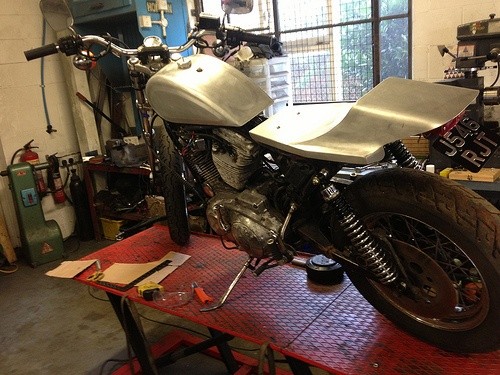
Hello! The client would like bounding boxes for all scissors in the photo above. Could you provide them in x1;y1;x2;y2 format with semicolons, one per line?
86;260;104;282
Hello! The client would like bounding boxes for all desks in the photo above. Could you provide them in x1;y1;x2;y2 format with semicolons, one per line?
69;222;500;375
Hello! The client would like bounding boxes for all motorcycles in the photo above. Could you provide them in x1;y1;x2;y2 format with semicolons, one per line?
23;0;500;353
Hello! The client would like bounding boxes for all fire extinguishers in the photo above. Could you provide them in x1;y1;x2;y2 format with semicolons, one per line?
47;152;66;204
11;139;46;198
